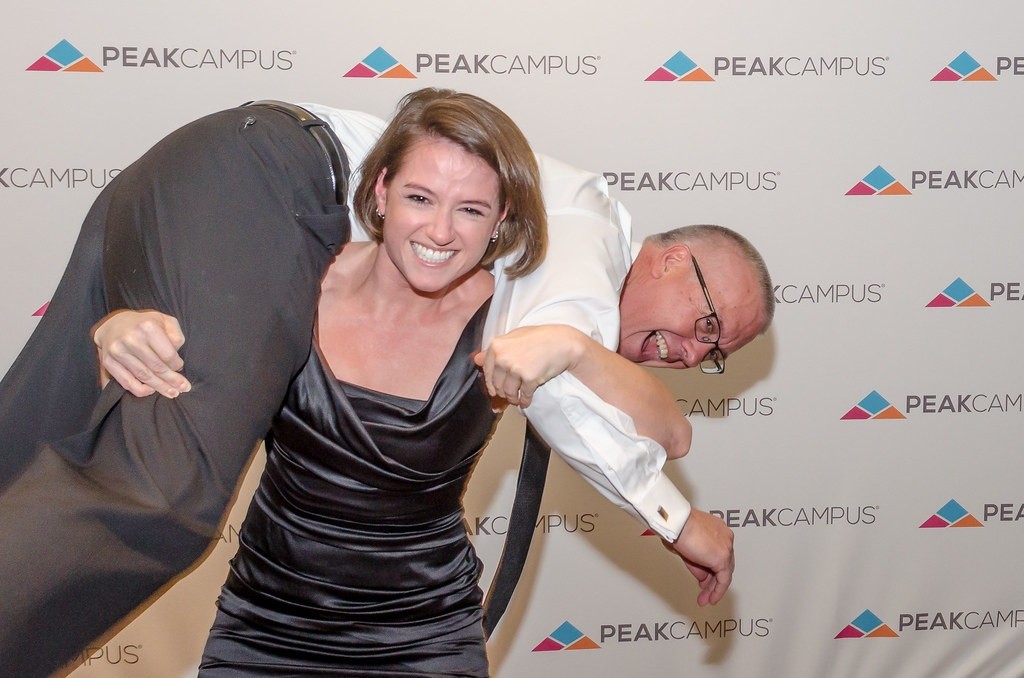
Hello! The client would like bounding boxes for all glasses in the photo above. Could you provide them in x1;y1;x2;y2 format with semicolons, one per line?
691;255;726;375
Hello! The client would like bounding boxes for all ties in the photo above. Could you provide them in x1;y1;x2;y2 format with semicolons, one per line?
481;418;552;640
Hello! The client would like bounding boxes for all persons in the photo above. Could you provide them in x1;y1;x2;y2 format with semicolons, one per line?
0;98;774;678
91;86;693;678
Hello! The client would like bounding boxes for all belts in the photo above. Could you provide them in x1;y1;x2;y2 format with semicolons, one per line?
246;100;347;206
